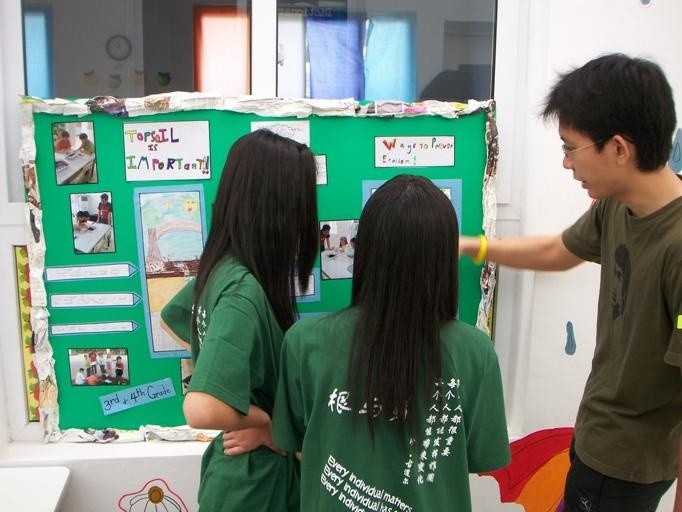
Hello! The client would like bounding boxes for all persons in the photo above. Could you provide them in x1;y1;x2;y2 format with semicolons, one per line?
72;194;112;231
55;131;94;155
159;129;319;512
75;349;124;385
319;224;356;254
459;54;681;512
271;176;512;512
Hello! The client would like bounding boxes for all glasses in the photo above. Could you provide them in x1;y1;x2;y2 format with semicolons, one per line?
561;133;634;160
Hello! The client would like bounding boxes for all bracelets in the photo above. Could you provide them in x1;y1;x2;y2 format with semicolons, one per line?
472;235;487;265
